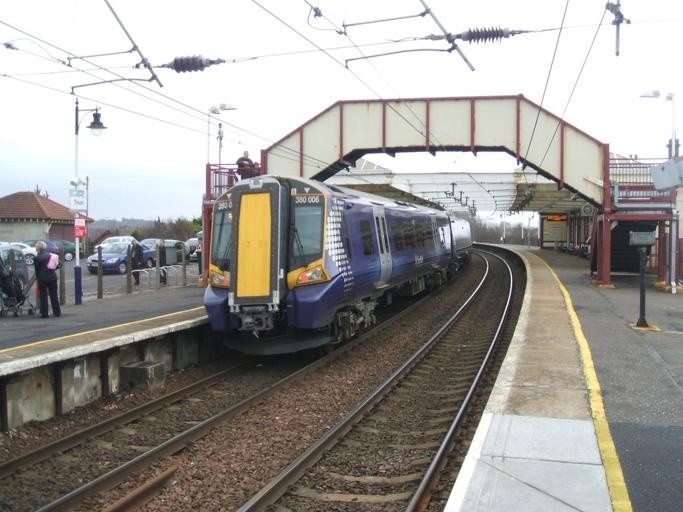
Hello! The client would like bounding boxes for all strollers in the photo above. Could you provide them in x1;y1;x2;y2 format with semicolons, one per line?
0;274;36;317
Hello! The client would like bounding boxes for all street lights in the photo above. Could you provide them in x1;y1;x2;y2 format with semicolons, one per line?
75;97;107;304
206;103;238;161
640;89;677;156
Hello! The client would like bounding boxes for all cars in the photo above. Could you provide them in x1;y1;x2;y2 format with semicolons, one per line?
86;236;198;274
0;239;84;289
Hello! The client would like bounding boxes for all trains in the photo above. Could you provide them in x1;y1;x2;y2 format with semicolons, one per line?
203;175;472;354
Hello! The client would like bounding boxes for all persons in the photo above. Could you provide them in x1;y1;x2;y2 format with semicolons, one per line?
157;238;167;285
235;151;254;181
253;162;260;177
32;240;63;320
129;239;144;286
